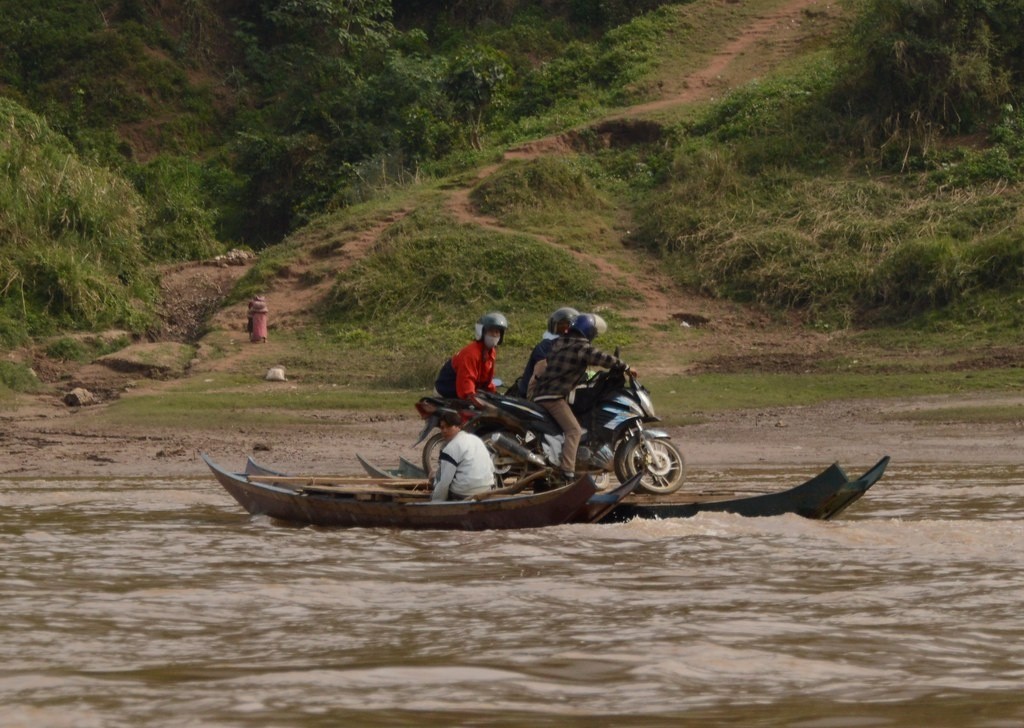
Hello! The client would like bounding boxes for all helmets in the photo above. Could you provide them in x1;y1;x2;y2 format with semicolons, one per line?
547;307;580;335
569;314;595;342
475;312;508;345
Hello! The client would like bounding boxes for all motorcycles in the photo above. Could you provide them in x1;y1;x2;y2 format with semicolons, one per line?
409;344;687;495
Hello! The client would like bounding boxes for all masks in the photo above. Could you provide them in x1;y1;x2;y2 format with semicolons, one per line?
483;335;501;348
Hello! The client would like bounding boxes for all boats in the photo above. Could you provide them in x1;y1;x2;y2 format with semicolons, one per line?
200;450;649;530
596;455;891;524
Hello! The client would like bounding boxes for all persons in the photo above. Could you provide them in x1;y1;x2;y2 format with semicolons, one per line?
531;313;639;483
519;305;580;400
432;411;495;503
434;314;507;410
